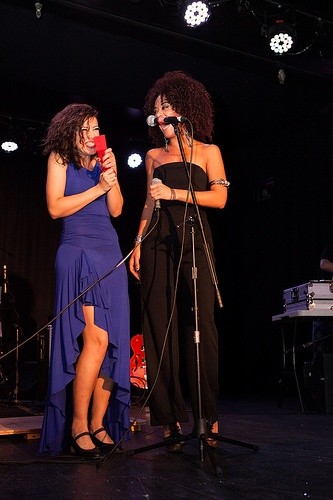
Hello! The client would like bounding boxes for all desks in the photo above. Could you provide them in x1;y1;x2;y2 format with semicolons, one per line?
271;310;333;414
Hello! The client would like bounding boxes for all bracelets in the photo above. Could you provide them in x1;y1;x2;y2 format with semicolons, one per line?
134;235;143;244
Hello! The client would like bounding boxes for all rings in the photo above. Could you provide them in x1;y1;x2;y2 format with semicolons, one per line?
112;177;114;181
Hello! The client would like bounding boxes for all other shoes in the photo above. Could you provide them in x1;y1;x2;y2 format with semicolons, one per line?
166;432;183;451
207;437;219;447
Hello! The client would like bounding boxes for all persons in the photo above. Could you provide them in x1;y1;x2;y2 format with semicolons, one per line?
312;245;333;368
30;104;130;458
129;73;227;446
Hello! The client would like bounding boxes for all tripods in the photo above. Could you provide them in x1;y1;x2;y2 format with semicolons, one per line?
124;122;261;477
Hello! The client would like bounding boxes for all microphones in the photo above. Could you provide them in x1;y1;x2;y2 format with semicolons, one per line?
151;178;162;209
147;115;187;127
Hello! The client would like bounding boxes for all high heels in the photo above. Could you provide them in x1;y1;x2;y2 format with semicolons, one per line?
89;428;120;454
68;432;102;459
